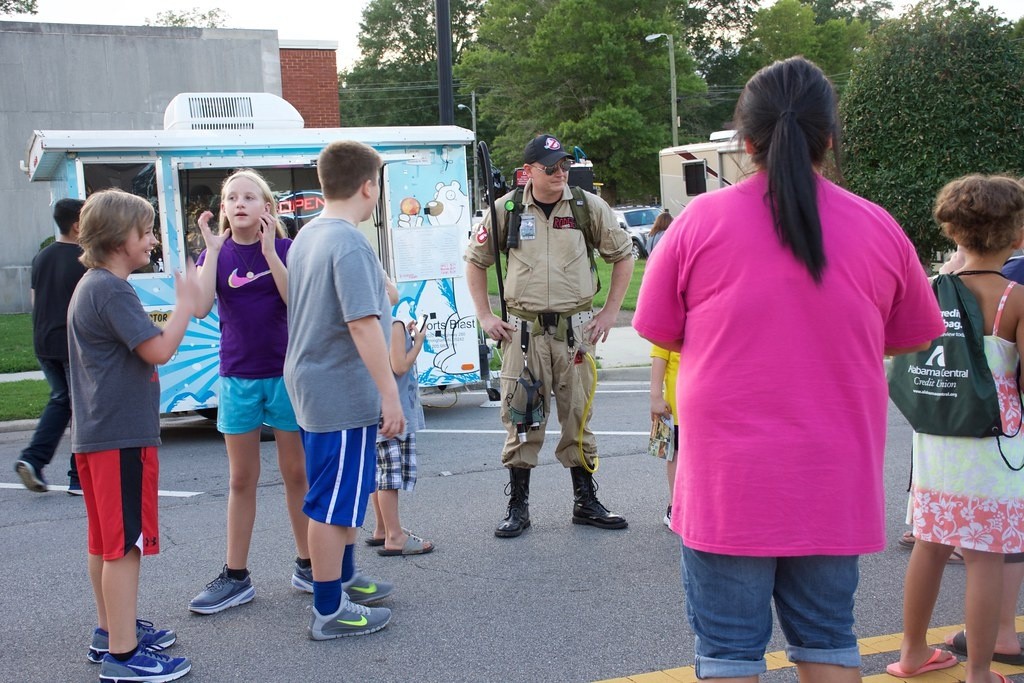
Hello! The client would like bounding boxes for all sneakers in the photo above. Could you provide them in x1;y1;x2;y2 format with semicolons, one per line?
68;475;84;496
188;564;254;614
292;560;313;593
13;456;48;493
99;644;191;683
307;592;391;640
87;620;176;663
342;575;394;603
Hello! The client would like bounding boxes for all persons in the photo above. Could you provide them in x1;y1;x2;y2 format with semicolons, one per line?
632;52;949;683
463;134;635;538
12;144;427;683
650;174;1024;683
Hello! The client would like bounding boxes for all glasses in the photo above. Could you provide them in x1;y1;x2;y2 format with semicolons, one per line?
532;160;571;175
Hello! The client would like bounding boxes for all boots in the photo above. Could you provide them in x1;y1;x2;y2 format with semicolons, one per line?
570;465;628;529
494;467;531;537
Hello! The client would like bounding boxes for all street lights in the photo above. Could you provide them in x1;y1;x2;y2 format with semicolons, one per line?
458;90;481;211
645;31;679;147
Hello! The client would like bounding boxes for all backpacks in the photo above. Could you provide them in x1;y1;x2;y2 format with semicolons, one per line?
887;273;1002;438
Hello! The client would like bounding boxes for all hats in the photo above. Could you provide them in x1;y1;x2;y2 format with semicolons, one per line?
524;134;575;167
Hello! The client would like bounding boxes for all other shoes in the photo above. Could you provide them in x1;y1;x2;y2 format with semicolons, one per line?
662;505;671;530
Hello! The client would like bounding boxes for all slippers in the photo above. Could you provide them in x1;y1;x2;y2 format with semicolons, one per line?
887;649;956;677
899;532;915;546
365;526;434;555
967;671;1013;683
946;630;1024;665
948;551;965;565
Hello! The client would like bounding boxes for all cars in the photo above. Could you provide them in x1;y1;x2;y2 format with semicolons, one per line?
608;204;664;261
